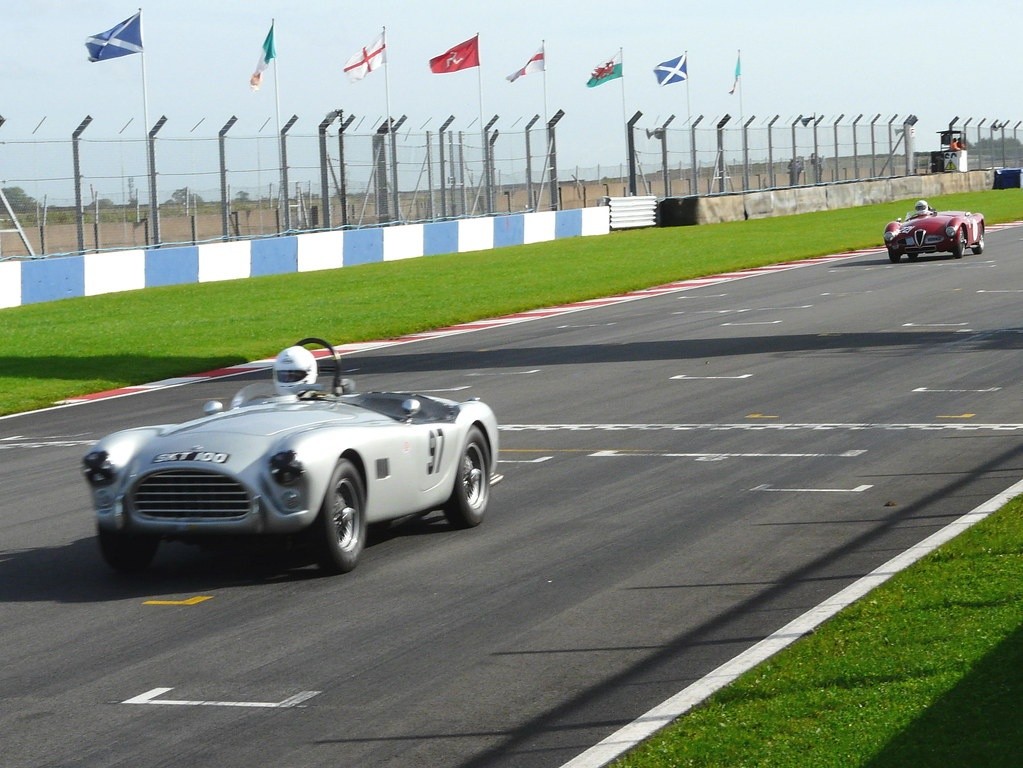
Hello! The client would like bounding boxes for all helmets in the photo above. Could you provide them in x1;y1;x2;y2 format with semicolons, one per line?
914;200;928;216
273;346;318;393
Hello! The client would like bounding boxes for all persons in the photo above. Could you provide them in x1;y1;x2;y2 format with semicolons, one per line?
951;138;960;150
957;138;965;150
915;200;928;216
273;345;318;397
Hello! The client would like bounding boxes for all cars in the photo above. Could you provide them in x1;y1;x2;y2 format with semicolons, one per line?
882;206;984;258
81;337;501;574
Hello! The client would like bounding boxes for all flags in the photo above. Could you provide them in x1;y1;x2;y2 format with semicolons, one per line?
342;30;387;82
728;55;741;95
250;26;277;94
653;54;689;87
505;42;546;83
586;51;623;87
84;12;143;63
429;36;481;74
